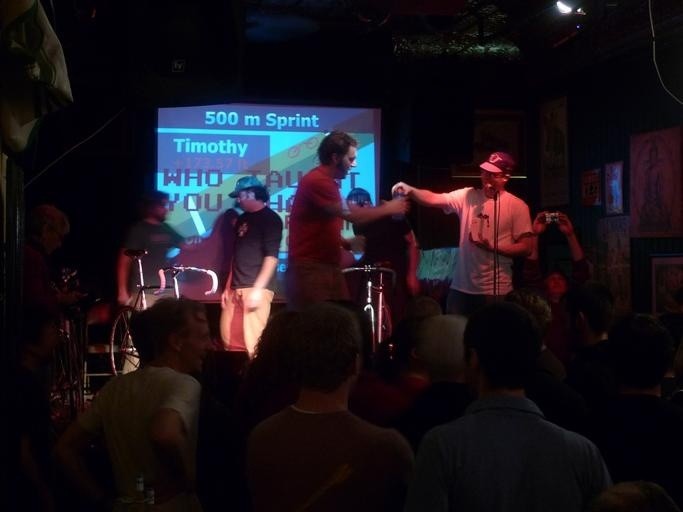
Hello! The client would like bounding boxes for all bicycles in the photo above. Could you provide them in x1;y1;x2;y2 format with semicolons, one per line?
46;267;88;437
338;260;398;354
107;246;218;378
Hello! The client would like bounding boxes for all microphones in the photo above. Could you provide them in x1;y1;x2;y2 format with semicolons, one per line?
123;248;148;257
484;183;498;195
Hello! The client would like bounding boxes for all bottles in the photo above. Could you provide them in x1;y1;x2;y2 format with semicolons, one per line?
391;188;405;221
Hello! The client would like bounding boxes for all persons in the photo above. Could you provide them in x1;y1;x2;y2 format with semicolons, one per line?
327;298;479;455
220;176;283;359
228;310;295;441
280;127;412;313
52;299;214;510
22;204;83;322
391;152;532;317
505;279;682;509
9;311;59;508
405;302;610;509
248;303;415;509
117;192;196;374
341;188;420;342
524;209;592;348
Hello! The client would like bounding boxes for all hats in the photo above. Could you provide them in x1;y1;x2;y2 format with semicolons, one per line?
228;176;266;198
479;151;514;176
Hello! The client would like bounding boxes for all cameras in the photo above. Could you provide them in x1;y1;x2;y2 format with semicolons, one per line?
544;211;560;226
